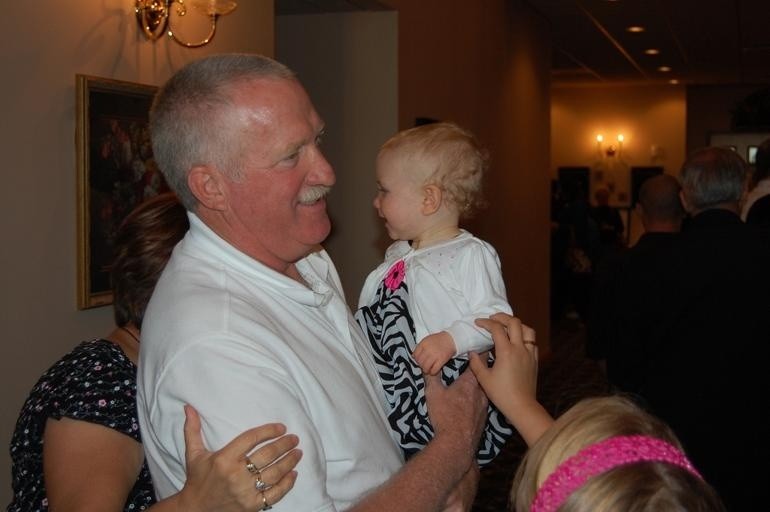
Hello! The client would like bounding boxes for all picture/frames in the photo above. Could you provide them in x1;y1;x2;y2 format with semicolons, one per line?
76;73;168;310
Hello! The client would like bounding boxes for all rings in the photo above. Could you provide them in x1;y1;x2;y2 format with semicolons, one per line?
522;339;537;347
260;491;273;511
245;456;260;475
255;473;266;493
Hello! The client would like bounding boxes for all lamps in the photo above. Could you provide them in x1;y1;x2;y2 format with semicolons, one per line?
596;134;625;160
134;0;237;48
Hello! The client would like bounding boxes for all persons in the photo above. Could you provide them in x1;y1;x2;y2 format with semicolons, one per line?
467;312;728;512
8;190;305;511
352;119;518;471
550;137;769;511
136;52;492;510
